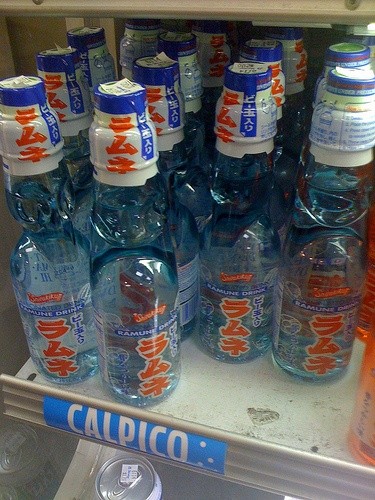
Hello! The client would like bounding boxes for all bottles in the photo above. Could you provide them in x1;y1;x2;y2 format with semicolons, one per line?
37;47;95;239
286;42;375;209
0;75;99;386
88;19;235;410
196;61;286;365
238;39;299;212
65;26;117;118
264;27;313;160
270;68;375;469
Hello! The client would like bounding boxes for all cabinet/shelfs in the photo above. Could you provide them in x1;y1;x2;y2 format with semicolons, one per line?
0;0;375;500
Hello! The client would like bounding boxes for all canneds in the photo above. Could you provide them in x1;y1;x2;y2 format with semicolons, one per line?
94;452;166;500
0;422;63;500
